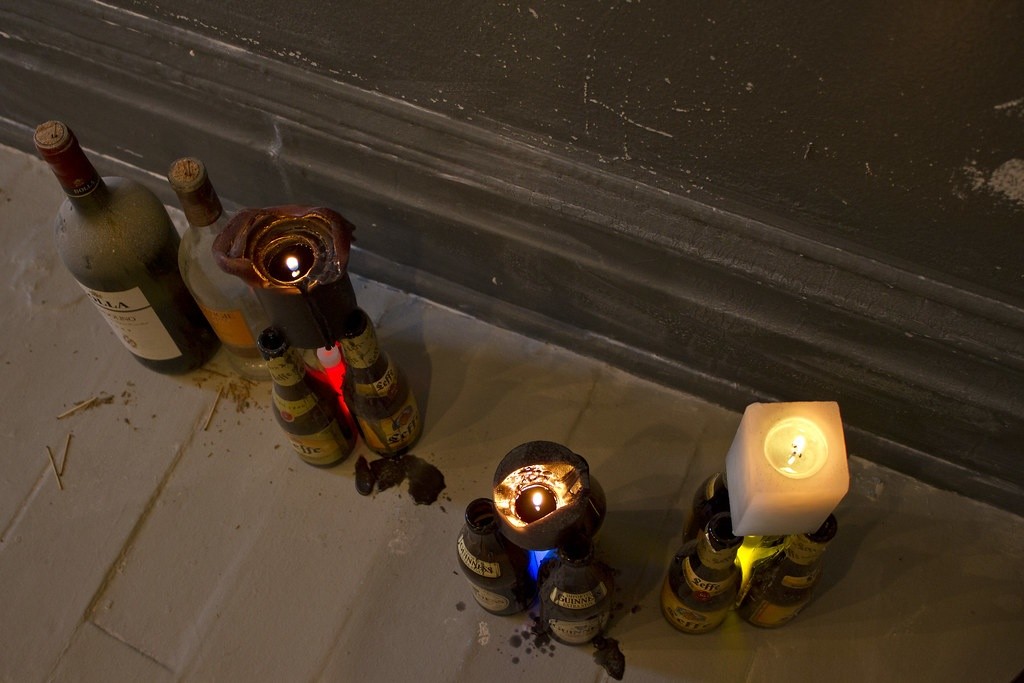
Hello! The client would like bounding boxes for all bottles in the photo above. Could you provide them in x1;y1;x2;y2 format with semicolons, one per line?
659;513;741;634
539;546;610;645
681;471;728;545
456;498;524;616
169;157;278;360
738;513;838;627
257;327;354;469
337;309;423;457
32;121;221;375
752;534;788;553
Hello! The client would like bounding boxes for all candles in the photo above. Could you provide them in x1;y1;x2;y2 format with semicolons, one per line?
725;401;849;536
